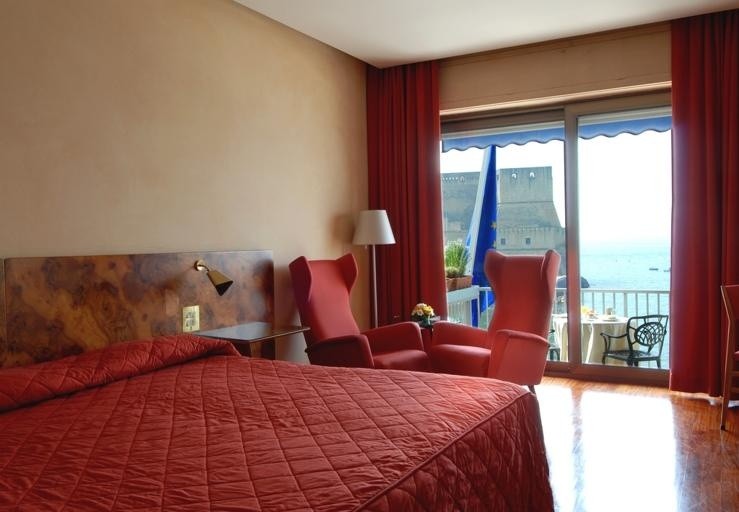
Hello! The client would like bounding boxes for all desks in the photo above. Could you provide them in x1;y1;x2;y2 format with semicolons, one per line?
189;321;311;359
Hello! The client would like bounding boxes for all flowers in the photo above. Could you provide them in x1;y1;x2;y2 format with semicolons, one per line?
410;302;435;327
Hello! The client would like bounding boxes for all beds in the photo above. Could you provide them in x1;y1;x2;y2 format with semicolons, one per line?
1;335;554;512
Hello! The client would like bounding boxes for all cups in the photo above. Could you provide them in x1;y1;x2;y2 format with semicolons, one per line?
605;307;616;320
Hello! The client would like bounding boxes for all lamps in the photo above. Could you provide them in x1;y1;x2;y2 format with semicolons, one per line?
350;209;397;329
195;259;234;296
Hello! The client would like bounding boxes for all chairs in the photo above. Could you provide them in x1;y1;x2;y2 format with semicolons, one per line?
428;248;560;400
599;314;669;368
287;251;428;371
719;284;738;430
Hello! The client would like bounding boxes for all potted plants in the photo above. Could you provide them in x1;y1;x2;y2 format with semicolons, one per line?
445;242;473;291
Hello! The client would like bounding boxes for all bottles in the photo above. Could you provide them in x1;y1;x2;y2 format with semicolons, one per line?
547;330;558;360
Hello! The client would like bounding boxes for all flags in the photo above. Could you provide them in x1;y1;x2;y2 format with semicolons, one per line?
459;144;498;329
